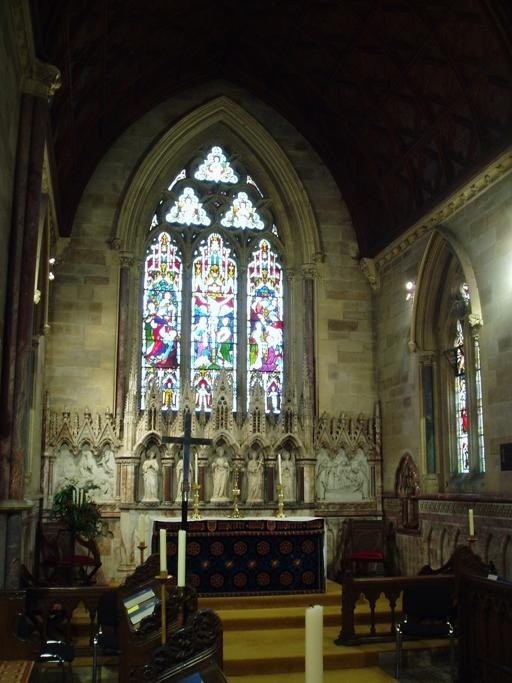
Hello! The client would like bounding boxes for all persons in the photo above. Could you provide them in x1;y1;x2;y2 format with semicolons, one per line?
174;450;193;501
141;450;160;502
279;451;296;498
54;445;115;501
317;446;373;503
246;450;264;500
147;288;282;409
211;446;229;496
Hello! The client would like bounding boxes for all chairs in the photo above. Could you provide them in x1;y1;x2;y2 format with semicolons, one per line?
92;589;121;683
394;581;456;681
26;639;77;681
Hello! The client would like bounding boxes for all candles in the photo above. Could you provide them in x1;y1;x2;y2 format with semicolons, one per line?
159;527;187;587
304;603;324;683
139;513;145;542
468;508;475;536
278;451;283;484
195;452;199;485
